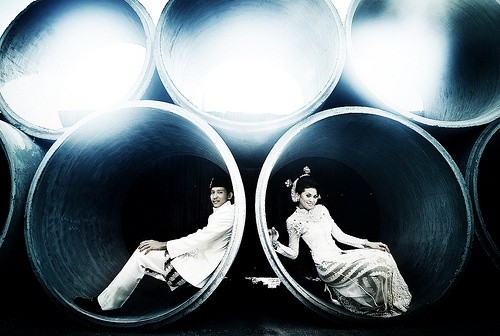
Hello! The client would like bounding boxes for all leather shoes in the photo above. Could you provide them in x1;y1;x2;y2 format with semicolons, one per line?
73;296;106;315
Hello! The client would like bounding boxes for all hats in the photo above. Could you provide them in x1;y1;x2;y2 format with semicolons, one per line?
210;176;231;187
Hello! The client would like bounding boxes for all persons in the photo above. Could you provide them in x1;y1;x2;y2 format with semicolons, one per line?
268;177;413;319
74;173;234;316
